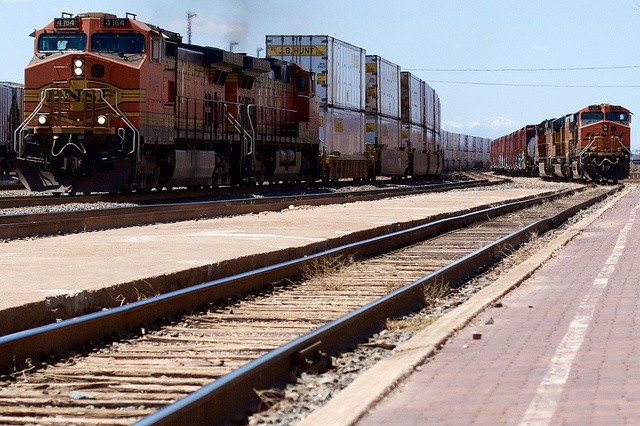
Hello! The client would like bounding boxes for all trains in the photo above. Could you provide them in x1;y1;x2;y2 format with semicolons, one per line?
0;83;25;177
491;105;632;184
14;12;492;196
